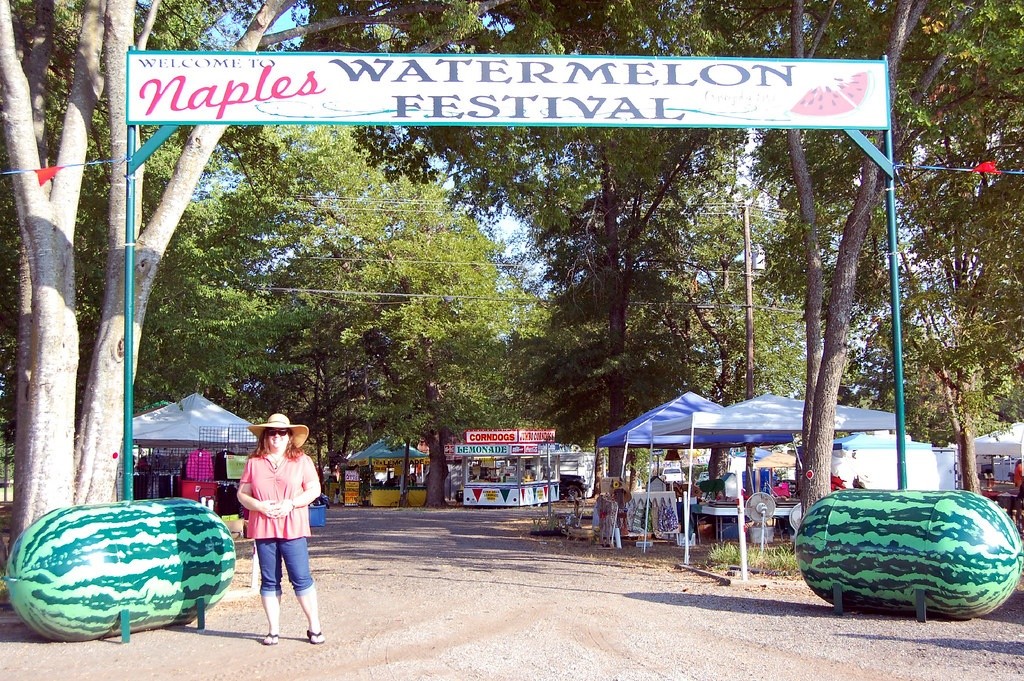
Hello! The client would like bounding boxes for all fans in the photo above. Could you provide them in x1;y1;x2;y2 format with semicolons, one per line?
790;503;802;530
745;492;776;551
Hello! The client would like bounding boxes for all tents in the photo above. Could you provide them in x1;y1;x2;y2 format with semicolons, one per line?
643;393;896;581
795;434;940;498
577;392;802;566
347;435;430;489
974;422;1024;484
116;392;259;503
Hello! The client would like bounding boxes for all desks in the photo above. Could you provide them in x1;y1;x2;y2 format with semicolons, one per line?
691;504;793;545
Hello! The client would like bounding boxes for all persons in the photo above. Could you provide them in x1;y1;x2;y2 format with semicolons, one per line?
237;412;325;644
410;473;417;486
1014;459;1024;500
468;460;474;476
485;467;491;481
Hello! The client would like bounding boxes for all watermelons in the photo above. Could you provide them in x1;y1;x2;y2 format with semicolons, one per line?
794;488;1024;619
4;496;235;641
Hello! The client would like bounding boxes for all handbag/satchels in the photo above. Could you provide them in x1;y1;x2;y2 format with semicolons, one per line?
626;497;678;539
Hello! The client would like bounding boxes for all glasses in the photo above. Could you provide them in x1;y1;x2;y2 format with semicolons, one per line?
267;429;288;436
264;633;279;645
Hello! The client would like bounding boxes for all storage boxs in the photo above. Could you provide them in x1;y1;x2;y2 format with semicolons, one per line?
309;505;326;526
749;527;775;544
677;533;696;547
722;522;738;540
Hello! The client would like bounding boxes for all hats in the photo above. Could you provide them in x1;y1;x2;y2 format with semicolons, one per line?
248;413;310;448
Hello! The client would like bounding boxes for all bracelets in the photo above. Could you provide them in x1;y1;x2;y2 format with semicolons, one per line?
291;501;295;510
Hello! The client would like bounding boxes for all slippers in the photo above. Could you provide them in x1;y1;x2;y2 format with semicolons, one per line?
307;629;325;644
